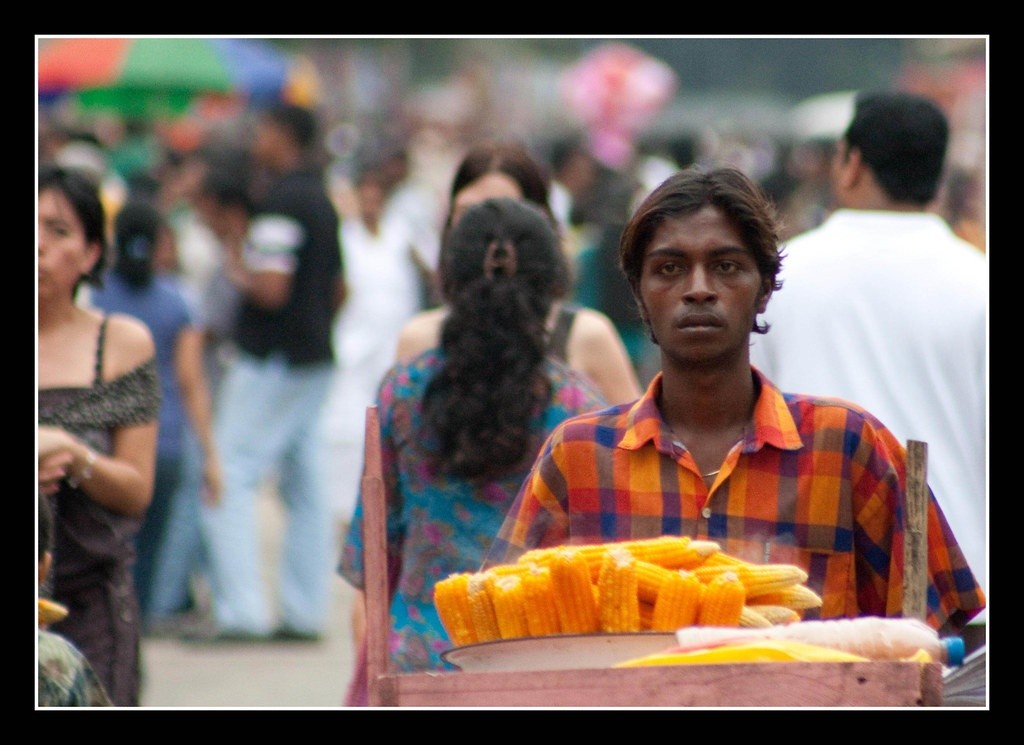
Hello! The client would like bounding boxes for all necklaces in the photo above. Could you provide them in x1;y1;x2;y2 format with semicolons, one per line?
703;469;720;479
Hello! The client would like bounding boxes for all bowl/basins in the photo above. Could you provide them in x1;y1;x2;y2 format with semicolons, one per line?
440;630;678;672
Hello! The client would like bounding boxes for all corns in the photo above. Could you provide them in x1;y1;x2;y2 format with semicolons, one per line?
433;536;823;647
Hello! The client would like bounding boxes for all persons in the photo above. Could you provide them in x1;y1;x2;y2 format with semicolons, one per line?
480;166;988;636
37;165;161;706
398;141;649;406
336;197;610;706
40;102;984;641
749;92;987;624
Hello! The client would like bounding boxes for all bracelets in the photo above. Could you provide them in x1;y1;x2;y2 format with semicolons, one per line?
69;454;95;488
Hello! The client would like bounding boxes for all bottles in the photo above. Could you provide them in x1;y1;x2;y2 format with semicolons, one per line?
770;616;965;670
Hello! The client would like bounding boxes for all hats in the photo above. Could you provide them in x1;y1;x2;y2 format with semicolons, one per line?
765;91;856;149
